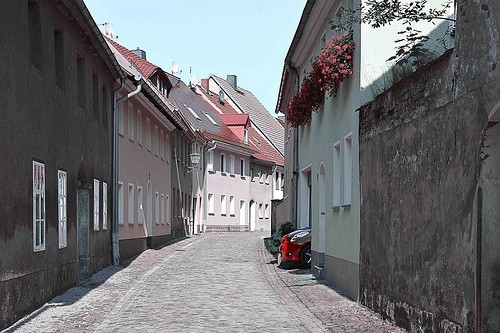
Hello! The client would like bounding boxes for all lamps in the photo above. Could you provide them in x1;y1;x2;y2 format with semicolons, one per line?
270;163;277;175
187;150;201;174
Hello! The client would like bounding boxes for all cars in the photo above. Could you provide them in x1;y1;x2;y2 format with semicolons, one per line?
278;227;311;269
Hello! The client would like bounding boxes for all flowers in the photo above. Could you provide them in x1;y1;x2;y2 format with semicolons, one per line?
284;32;354;128
